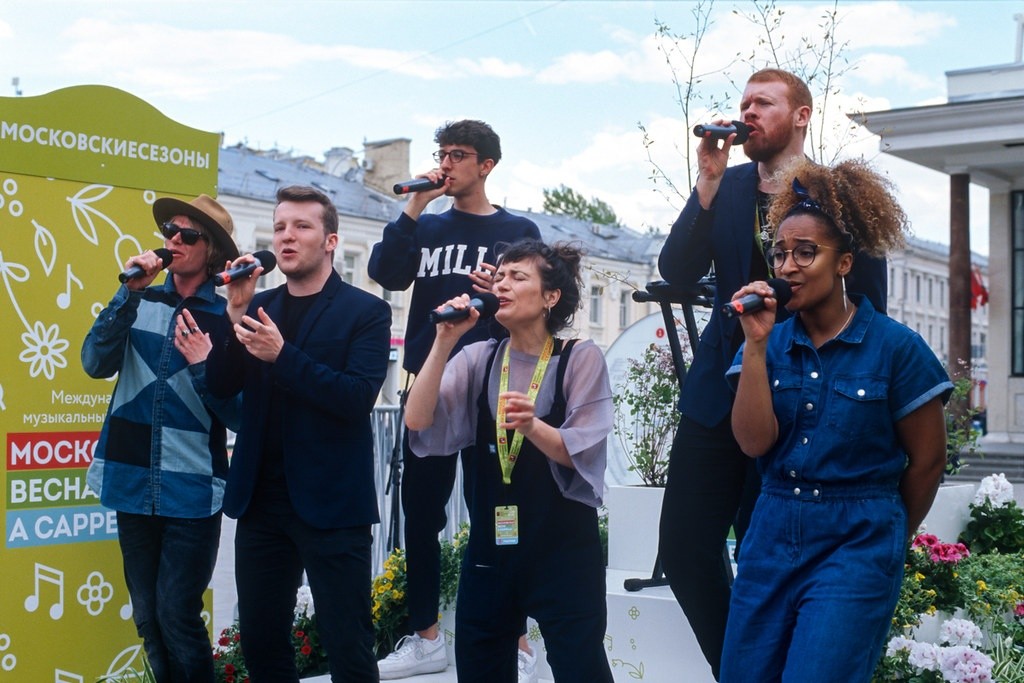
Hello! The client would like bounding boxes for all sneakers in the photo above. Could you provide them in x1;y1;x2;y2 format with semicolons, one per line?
518;646;539;683
376;631;448;679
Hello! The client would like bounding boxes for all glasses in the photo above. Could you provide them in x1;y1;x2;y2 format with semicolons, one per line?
162;222;209;245
433;149;478;164
765;242;846;270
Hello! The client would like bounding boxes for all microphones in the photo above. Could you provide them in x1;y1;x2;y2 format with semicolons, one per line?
213;251;276;287
428;293;500;324
119;249;173;284
393;174;446;195
693;120;750;144
722;280;792;317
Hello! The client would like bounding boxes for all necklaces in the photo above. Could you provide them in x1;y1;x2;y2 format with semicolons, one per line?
833;303;855;338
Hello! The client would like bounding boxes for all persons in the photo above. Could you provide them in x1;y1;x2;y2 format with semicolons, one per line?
656;68;889;683
366;120;540;682
404;237;617;683
719;150;947;683
202;189;392;683
81;198;231;683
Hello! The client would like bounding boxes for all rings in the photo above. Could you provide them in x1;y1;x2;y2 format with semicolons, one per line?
182;330;190;338
191;327;199;334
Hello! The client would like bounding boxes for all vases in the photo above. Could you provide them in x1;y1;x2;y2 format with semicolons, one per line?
608;484;665;571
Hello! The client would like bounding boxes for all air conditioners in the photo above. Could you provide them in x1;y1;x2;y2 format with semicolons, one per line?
361;159;375;172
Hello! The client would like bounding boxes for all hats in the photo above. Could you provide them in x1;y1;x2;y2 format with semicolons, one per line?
152;193;241;264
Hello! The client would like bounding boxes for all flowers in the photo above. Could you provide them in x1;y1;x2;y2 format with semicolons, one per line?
611;335;694;486
